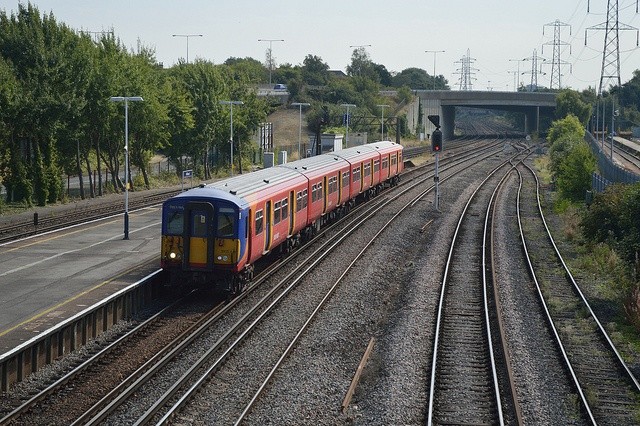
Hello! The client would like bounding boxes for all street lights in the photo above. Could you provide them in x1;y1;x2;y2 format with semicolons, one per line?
350;45;371;53
258;39;284;86
77;30;112;44
376;104;390;140
292;103;311;161
340;104;357;149
222;100;245;178
425;50;445;90
111;97;145;239
172;34;204;64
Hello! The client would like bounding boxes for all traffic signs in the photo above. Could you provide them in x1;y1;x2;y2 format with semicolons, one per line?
184;171;192;177
344;114;351;125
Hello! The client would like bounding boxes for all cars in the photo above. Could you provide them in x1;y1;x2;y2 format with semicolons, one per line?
273;83;288;91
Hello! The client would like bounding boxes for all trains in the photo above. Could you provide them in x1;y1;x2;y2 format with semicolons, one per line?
161;141;403;302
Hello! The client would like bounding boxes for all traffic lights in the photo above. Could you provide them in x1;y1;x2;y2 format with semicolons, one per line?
428;114;439;126
432;130;442;151
